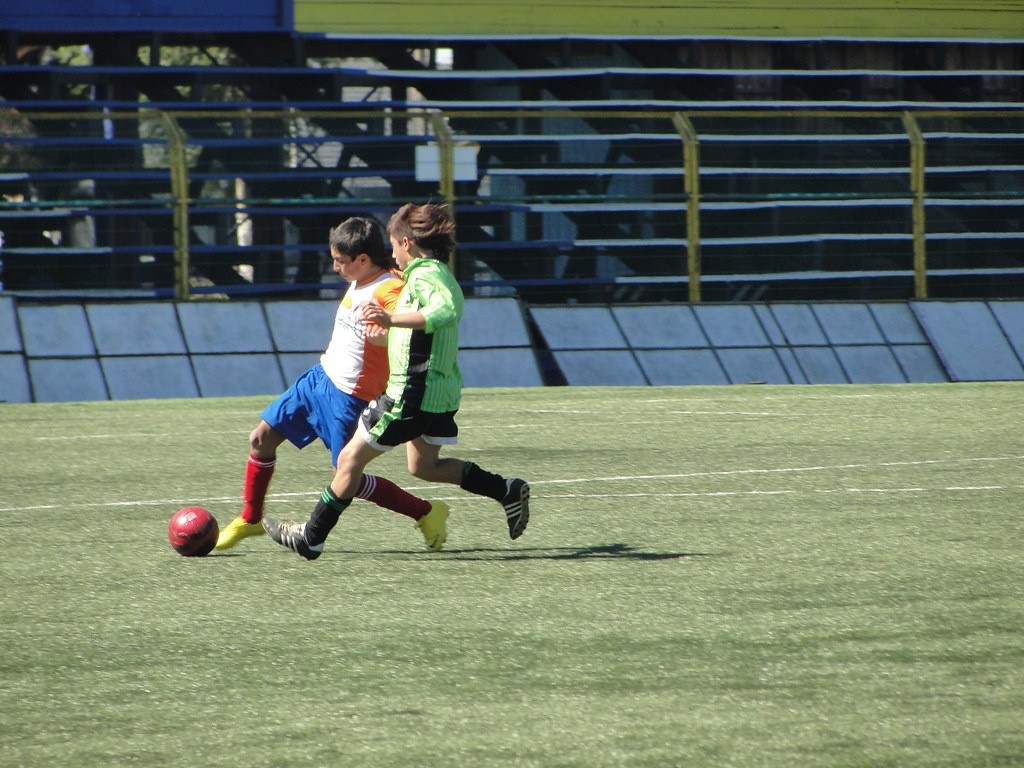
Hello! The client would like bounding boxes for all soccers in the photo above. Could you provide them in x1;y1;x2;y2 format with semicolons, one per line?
168;507;219;557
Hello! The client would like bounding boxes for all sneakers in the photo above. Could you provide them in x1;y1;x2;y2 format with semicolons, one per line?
215;517;267;550
500;478;529;540
410;500;450;551
261;516;324;561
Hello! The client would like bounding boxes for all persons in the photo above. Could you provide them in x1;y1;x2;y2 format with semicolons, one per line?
261;204;531;561
216;216;450;552
